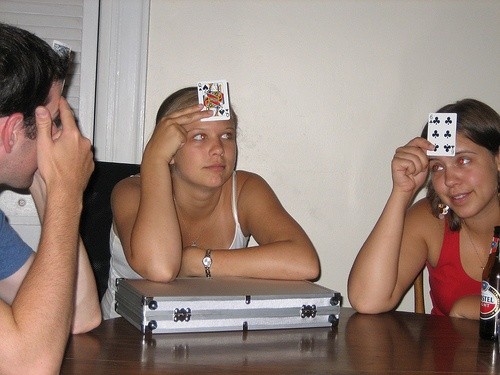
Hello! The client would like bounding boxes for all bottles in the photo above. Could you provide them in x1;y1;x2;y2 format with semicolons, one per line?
480;226;500;341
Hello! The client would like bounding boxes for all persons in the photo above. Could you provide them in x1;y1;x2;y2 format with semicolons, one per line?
102;88;322;319
347;99;500;320
0;23;103;375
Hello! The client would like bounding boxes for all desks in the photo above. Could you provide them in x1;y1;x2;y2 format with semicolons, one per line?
58;306;500;375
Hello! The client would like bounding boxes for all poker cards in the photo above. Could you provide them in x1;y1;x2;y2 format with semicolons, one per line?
52;39;73;96
426;112;457;157
198;80;232;122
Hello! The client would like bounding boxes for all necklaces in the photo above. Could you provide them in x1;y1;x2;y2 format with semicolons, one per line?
173;200;200;248
464;230;485;269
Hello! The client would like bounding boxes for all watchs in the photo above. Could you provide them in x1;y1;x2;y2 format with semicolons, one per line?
202;248;212;277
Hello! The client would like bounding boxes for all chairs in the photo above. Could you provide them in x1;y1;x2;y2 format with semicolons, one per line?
78;160;141;301
